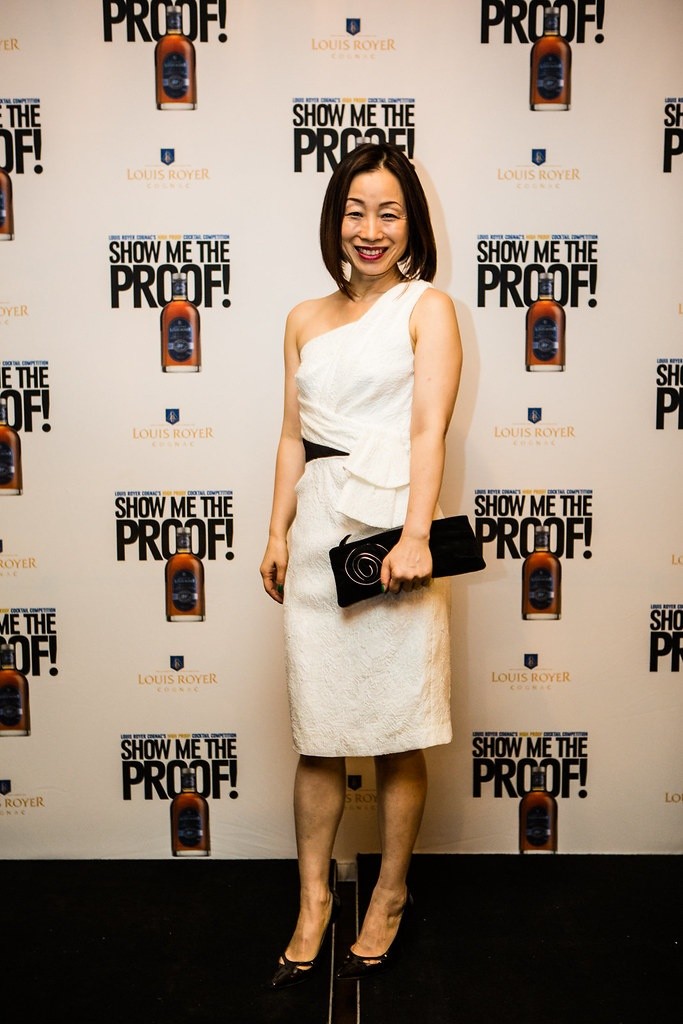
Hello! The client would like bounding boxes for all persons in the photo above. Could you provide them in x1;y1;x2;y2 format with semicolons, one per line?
262;145;464;986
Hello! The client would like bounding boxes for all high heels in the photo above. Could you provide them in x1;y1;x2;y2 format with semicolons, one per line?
271;891;342;987
337;888;413;979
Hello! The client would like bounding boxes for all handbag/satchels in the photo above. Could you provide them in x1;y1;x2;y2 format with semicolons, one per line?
329;515;486;608
